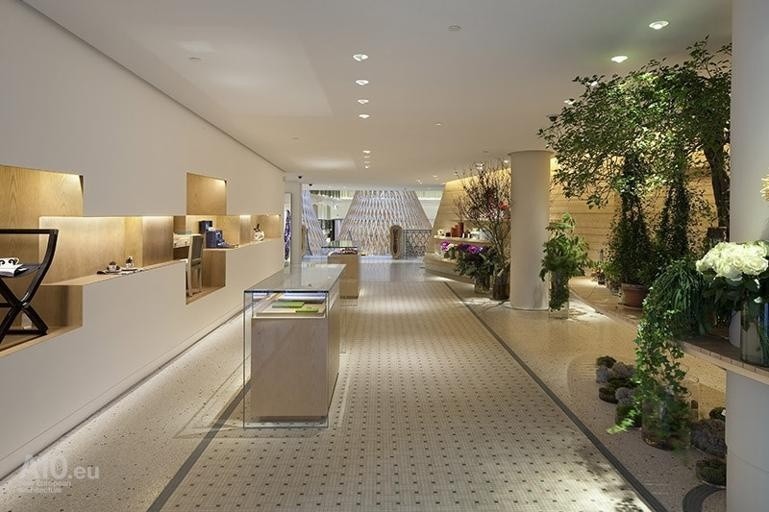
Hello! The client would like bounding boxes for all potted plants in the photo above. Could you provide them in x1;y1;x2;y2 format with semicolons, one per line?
451;157;517;300
538;211;602;319
601;183;667;309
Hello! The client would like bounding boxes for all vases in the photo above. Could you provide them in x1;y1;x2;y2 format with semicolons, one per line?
726;310;742;348
473;277;491;295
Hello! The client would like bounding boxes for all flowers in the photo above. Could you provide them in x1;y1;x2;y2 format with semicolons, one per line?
440;240;497;285
695;239;769;311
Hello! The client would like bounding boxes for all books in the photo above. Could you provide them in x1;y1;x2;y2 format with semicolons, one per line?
272;292;324;314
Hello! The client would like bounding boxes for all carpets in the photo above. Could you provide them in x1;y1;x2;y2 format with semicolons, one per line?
143;280;667;512
